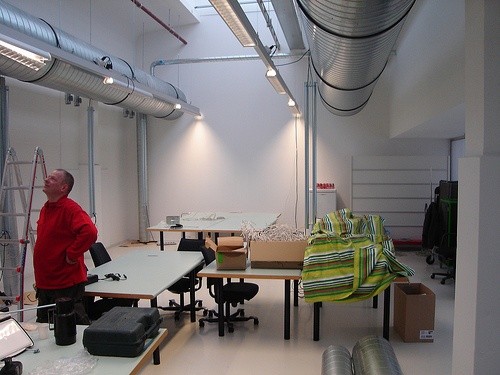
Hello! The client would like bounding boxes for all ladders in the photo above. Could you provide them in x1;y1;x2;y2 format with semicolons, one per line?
0;146;47;322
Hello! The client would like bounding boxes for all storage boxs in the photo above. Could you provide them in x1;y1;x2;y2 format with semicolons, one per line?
205;236;248;270
394;283;436;342
83;307;162;357
250;240;308;269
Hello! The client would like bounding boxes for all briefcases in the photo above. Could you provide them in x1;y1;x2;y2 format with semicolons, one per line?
83;307;164;358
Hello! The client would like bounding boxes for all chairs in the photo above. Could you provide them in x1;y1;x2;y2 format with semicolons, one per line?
82;264;133;321
90;242;140;307
430;231;456;284
199;245;259;332
157;238;208;317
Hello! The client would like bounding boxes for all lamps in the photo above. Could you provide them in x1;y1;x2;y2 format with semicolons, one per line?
99;56;113;70
103;77;153;99
288;99;300;116
173;104;202;120
265;70;286;95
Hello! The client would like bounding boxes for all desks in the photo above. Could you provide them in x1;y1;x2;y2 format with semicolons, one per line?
2;211;408;375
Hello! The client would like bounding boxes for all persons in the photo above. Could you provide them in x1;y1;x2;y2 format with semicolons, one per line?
33;169;97;325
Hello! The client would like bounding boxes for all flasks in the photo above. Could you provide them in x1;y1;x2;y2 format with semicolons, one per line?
48;296;77;347
